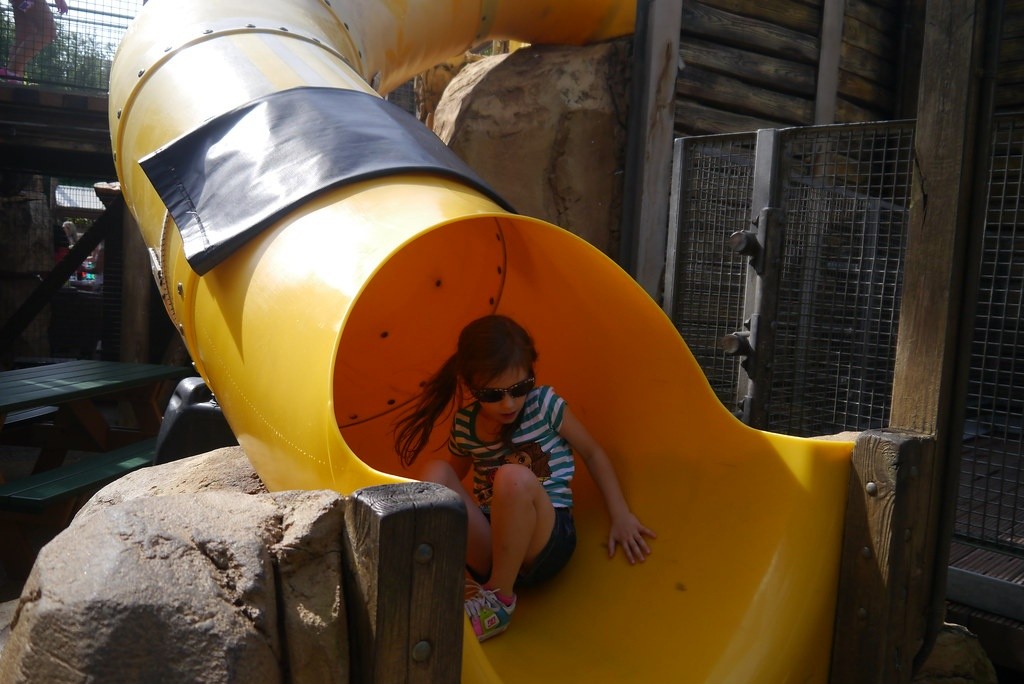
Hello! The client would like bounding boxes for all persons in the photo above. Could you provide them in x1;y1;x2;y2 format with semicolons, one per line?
391;315;658;643
52;219;105;288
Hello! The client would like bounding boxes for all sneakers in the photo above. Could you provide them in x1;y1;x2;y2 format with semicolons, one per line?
460;558;479;604
465;587;517;643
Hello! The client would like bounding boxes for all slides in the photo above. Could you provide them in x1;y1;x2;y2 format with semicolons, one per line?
107;0;858;684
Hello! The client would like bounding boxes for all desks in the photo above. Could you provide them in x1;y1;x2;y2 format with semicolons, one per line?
0;359;194;582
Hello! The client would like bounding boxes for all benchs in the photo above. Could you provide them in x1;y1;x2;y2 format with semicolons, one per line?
3;405;64;425
0;437;157;511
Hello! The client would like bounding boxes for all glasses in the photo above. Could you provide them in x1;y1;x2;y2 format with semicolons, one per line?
465;362;537;404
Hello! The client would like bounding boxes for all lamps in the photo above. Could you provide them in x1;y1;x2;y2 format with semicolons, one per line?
722;330;753;359
729;230;757;255
